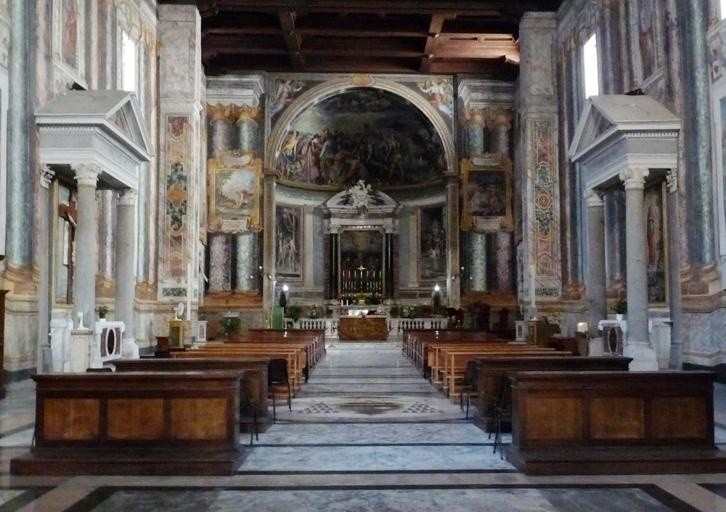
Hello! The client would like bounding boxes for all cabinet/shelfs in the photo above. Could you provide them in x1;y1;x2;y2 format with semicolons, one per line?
472;356;633;433
512;369;713;460
106;359;275;435
12;372;253;473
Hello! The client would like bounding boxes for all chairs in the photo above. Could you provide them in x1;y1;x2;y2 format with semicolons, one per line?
487;381;512;459
460;360;479;421
240;379;259;447
265;359;292;424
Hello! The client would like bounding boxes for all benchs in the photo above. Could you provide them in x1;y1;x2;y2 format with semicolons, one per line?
168;328;329;402
405;332;573;403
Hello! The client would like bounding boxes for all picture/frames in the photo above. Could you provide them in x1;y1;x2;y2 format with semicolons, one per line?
207;149;262;234
460;153;513;233
639;176;670;308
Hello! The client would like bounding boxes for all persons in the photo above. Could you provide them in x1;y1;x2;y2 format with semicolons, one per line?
278;127;447;185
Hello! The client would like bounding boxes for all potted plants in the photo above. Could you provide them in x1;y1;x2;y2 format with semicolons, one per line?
95;303;109;322
610;299;627;320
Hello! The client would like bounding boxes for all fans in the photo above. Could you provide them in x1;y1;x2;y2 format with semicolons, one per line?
173;301;184;321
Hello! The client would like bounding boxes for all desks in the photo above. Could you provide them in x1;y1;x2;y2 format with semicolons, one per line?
340;315;388;340
